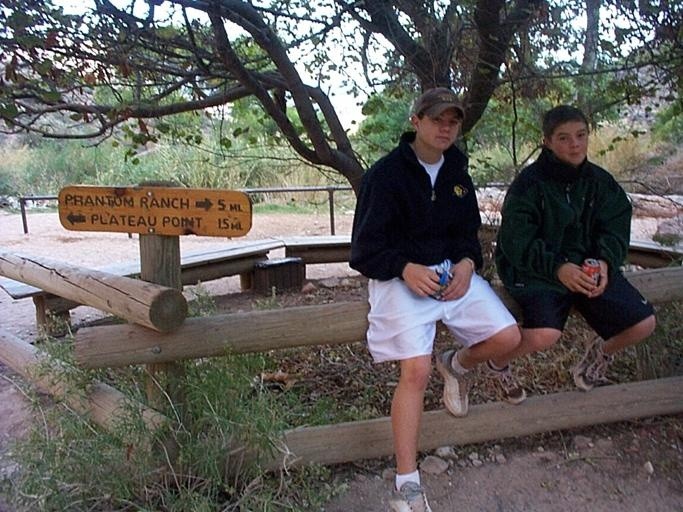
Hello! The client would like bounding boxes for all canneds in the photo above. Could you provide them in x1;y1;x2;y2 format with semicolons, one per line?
582;258;601;286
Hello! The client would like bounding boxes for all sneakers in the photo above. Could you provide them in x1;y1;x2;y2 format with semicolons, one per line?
436;350;472;418
480;357;527;405
572;336;611;392
387;481;433;512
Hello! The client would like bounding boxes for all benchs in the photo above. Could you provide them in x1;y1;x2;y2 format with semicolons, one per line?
0;238;283;340
283;234;352;265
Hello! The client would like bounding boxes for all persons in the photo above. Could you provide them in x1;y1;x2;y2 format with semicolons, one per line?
483;104;657;406
346;86;522;511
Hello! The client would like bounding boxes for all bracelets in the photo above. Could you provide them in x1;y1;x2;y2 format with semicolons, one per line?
462;256;476;274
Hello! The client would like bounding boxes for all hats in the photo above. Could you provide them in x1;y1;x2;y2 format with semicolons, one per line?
414;87;466;121
